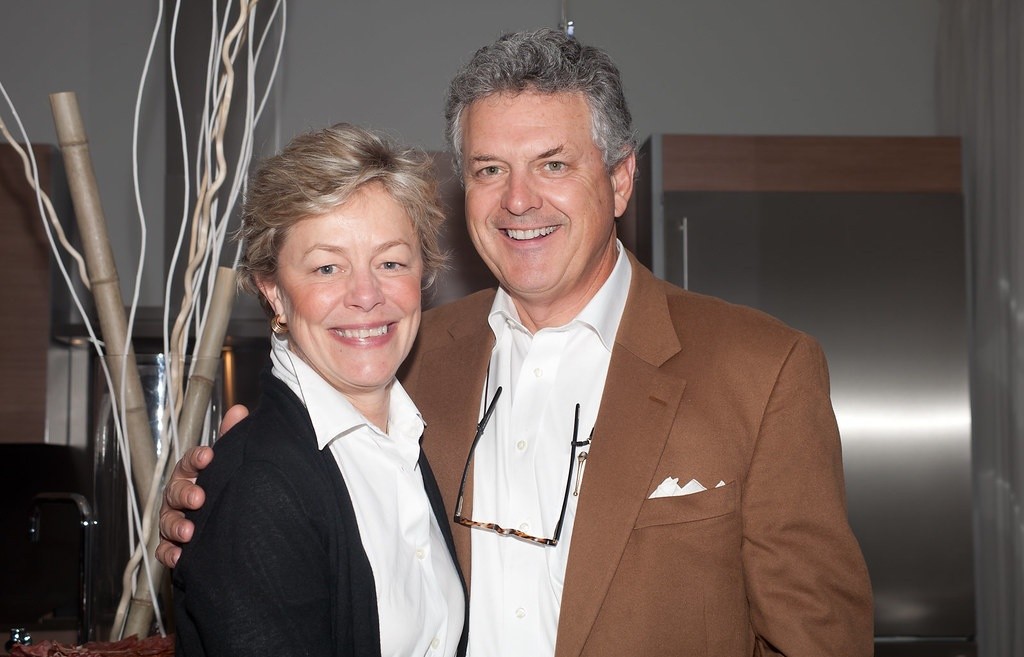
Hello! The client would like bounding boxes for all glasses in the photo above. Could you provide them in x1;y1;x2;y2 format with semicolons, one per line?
453;387;580;548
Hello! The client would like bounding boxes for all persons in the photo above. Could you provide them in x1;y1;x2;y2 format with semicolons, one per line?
156;123;470;657
156;29;875;657
